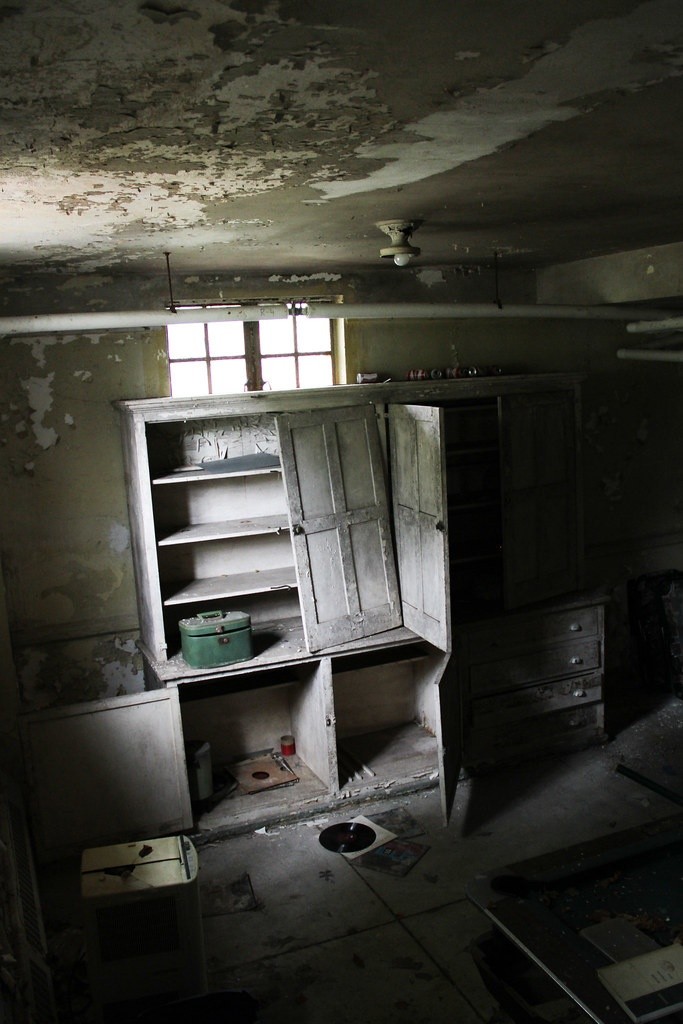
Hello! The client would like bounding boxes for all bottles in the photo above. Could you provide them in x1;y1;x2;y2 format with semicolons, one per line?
280;735;295;755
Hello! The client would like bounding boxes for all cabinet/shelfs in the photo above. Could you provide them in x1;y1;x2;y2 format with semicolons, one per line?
18;368;613;868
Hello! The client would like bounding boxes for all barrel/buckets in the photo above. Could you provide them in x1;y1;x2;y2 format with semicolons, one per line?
184;738;213;801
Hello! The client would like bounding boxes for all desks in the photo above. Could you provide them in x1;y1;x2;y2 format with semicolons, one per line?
464;813;683;1024
